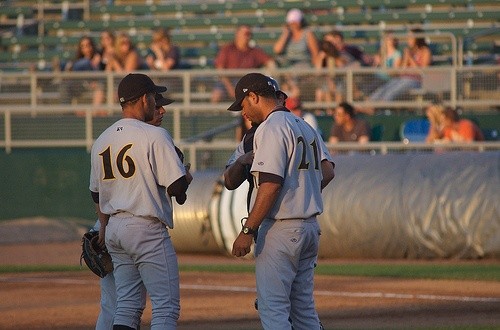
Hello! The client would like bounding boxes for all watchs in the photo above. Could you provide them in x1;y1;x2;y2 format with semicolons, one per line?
241;227;255;235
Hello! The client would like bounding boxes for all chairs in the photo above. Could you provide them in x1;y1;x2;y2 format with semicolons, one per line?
0;0;498;142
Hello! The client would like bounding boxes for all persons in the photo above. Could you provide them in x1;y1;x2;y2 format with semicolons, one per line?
89;74;192;330
89;93;183;330
56;8;483;145
223;72;335;330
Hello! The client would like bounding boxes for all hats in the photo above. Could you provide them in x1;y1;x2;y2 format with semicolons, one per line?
226;73;273;111
264;75;288;101
154;93;175;108
118;73;168;103
284;8;303;24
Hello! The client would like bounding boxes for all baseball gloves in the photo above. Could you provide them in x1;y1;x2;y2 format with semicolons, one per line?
79;231;115;279
